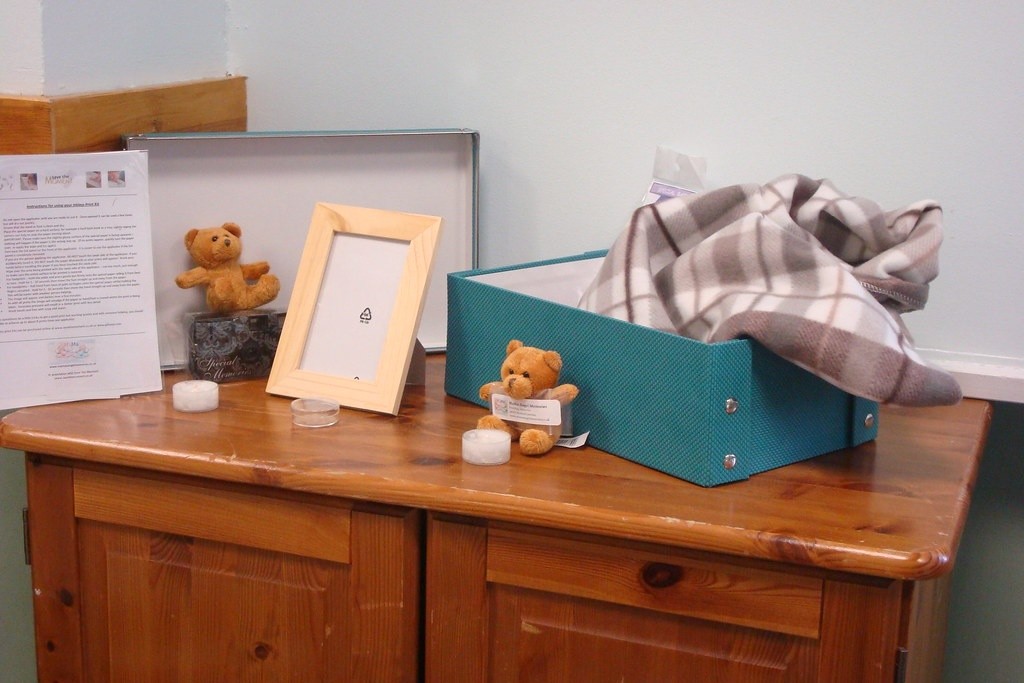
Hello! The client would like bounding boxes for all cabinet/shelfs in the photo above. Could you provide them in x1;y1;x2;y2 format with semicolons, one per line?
2;347;992;683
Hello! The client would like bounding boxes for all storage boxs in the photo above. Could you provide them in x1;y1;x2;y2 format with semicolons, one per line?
445;246;882;490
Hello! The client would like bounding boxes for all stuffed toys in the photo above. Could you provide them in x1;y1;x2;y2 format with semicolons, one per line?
479;340;579;455
175;222;281;315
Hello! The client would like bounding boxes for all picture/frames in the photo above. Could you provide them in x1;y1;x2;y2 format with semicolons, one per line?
264;203;445;417
